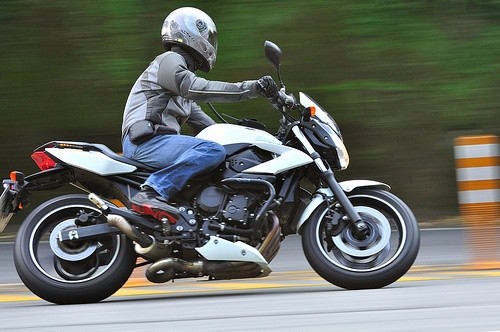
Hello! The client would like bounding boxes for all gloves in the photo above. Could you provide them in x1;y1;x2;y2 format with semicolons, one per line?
256;75;278;101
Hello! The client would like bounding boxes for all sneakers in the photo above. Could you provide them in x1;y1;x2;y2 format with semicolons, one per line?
130;186;179;224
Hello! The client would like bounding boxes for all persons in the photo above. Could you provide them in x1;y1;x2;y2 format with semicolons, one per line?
118;8;276;224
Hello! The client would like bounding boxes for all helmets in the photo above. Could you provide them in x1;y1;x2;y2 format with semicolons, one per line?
161;7;218;73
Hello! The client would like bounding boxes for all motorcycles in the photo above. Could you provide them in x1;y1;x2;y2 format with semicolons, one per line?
0;41;421;307
